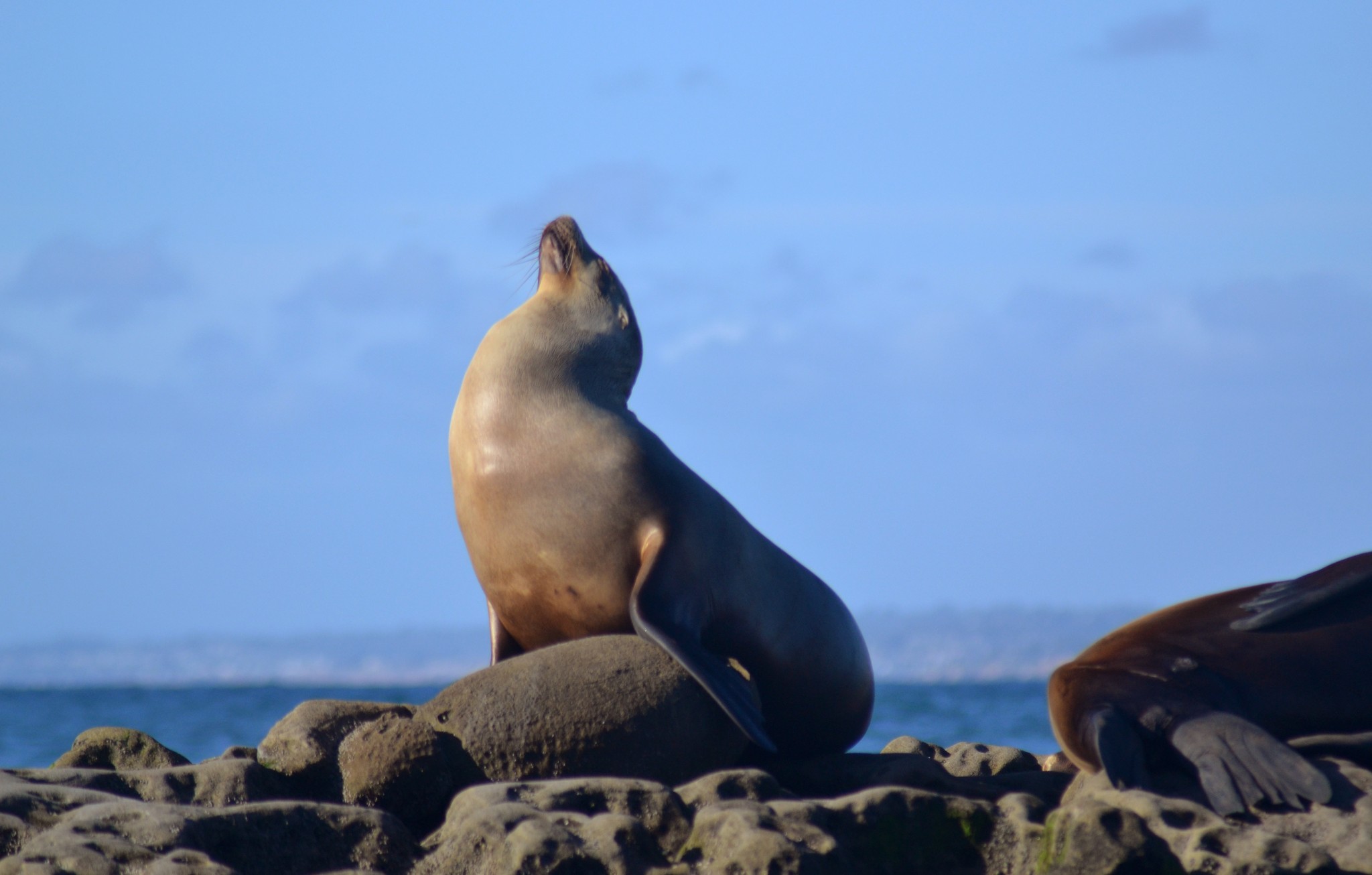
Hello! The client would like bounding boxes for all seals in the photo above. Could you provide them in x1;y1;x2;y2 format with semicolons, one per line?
445;211;878;763
